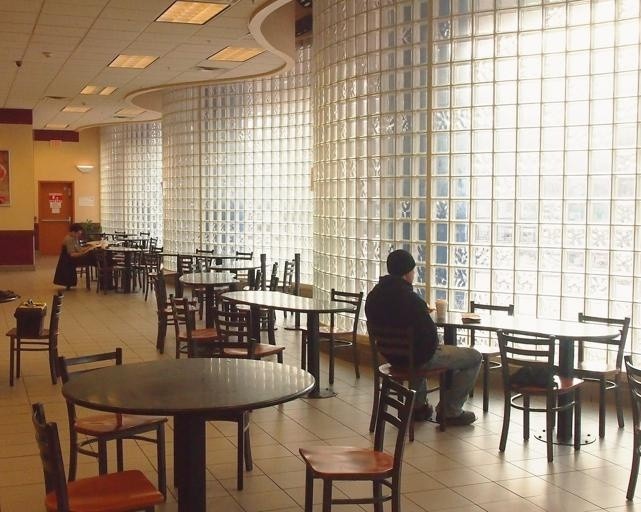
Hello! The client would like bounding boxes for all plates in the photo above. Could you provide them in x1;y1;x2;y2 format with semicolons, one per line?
461;312;481;323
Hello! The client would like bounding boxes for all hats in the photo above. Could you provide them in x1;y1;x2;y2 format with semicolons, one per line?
387;249;415;276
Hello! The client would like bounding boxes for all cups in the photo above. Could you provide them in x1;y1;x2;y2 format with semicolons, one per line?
435;299;448;322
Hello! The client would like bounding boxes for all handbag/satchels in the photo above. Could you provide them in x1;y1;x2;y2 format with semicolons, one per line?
510;365;558;390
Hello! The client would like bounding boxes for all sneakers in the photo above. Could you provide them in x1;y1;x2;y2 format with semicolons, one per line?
436;409;475;426
414;403;432;421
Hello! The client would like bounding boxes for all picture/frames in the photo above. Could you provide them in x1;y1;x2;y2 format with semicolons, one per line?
0;149;12;207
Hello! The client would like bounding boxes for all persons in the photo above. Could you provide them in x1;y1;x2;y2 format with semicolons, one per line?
61;222;116;291
365;250;483;427
